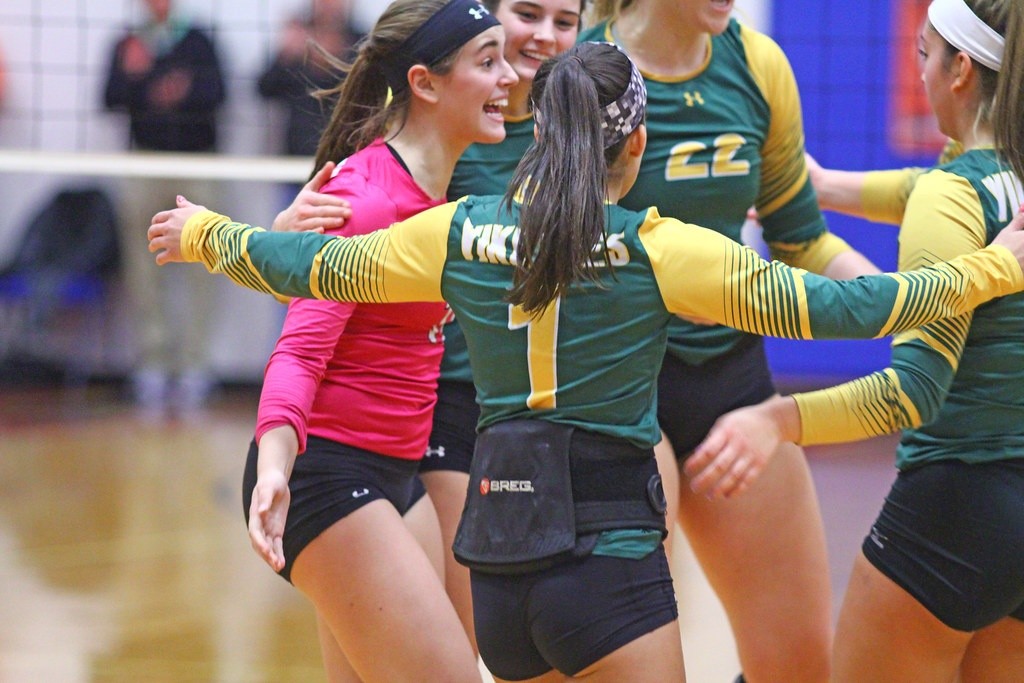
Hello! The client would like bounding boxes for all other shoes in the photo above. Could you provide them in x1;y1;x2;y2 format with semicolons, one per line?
135;365;219;413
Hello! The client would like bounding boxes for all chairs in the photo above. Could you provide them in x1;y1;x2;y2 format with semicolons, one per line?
0;188;122;387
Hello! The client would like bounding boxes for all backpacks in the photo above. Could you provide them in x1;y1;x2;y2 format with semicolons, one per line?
3;188;121;278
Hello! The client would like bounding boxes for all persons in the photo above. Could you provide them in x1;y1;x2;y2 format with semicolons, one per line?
0;0;1024;683
145;44;1024;683
